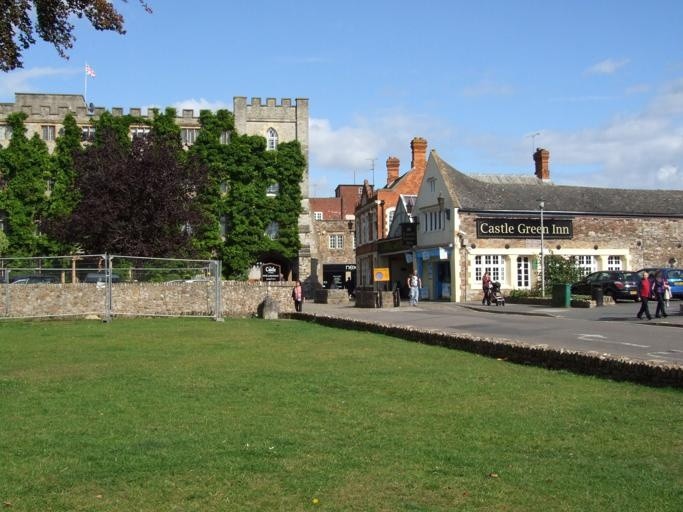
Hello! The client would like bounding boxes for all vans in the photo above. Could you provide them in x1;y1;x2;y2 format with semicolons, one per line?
85;273;121;283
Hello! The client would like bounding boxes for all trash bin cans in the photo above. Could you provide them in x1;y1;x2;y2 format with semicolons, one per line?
552;283;571;308
591;287;603;306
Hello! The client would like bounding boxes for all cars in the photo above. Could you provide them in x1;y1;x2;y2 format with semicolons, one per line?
9;276;60;284
570;267;683;302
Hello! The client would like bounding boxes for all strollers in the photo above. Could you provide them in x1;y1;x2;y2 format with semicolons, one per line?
487;280;505;306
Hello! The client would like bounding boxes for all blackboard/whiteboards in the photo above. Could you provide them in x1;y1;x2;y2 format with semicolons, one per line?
260;263;281;281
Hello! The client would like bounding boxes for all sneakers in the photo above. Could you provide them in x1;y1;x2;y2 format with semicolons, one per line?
637;313;668;320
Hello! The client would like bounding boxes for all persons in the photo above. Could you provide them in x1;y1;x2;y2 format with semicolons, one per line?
664;282;672;308
636;272;652;320
346;277;353;294
653;270;668;318
292;280;305;312
482;271;492;306
407;270;422;306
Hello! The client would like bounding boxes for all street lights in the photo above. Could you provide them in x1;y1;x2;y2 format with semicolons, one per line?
536;196;546;298
